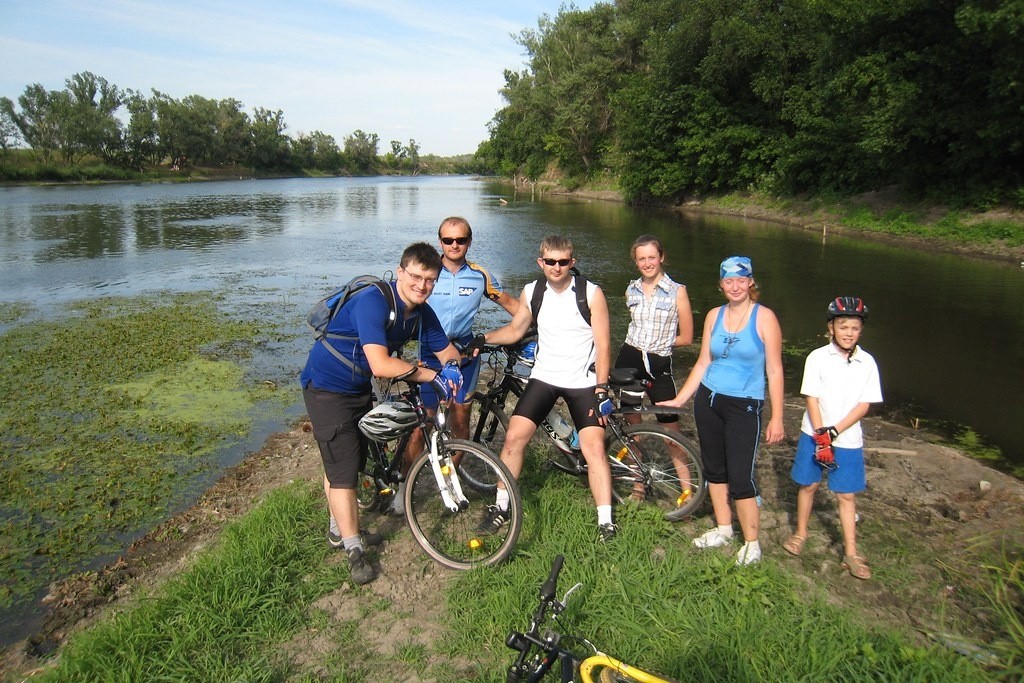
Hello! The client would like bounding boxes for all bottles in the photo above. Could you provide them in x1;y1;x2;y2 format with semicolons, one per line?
546;407;572;440
569;425;581;450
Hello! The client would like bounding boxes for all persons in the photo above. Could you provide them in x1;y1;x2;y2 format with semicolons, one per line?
300;241;464;585
655;257;784;567
614;234;693;522
781;297;883;579
465;235;619;545
387;216;537;515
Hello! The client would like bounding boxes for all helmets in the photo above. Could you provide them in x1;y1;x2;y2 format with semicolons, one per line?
357;401;421;444
828;296;869;321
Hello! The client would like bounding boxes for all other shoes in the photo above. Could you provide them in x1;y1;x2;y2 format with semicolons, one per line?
629;486;646;503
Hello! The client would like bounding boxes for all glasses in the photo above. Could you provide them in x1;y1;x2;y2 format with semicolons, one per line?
541;257;572;266
405;269;437;286
440;237;469;245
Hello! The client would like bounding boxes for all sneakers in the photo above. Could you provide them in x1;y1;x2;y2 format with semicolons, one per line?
692;527;735;548
326;532;343;549
385;482;405;517
734;545;762;569
475;503;511;536
596;523;616;544
345;546;374;586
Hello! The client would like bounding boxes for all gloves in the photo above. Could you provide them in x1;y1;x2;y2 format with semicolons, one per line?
812;427;838;462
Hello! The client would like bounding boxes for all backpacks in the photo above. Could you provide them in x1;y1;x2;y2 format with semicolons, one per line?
306;270;399;358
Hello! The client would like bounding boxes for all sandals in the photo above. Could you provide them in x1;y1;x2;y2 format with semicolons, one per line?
782;534;807;556
841;555;871;580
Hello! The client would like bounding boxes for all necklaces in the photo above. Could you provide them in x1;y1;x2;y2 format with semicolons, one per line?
729;303;750;344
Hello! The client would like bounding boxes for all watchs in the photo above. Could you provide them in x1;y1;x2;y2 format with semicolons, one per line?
446;359;459;368
595;383;609;391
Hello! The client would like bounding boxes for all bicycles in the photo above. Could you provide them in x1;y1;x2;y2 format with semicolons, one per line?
502;553;672;683
356;361;524;572
442;328;708;521
